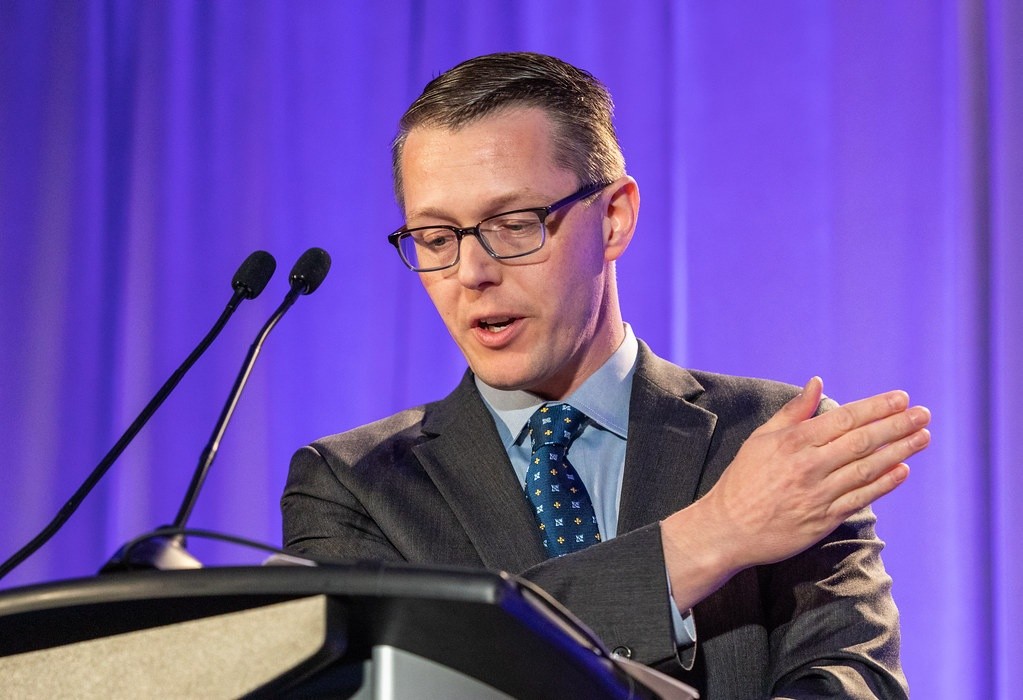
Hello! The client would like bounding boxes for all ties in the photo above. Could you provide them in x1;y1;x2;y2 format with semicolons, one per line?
523;404;603;564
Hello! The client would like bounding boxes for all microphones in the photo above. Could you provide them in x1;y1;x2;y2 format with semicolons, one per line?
0;248;275;580
98;247;333;568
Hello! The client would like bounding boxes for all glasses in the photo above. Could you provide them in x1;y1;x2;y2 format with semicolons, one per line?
388;178;616;272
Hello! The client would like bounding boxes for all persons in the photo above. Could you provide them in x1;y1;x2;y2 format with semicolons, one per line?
277;53;934;700
